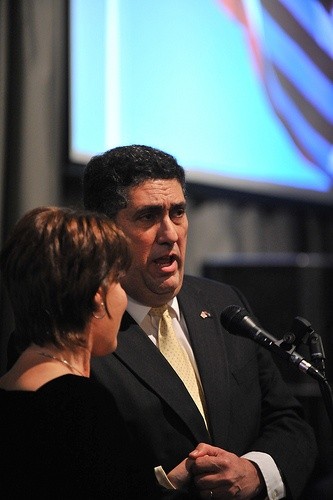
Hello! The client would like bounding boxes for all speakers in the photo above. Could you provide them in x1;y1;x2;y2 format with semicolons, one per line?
200;254;332;395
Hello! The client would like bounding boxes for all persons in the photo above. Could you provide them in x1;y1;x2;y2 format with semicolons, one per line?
0;207;209;500
7;145;319;500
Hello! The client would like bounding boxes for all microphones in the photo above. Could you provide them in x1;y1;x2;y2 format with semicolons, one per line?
220;303;327;389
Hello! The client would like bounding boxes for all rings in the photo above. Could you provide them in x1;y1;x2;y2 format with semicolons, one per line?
210;490;213;498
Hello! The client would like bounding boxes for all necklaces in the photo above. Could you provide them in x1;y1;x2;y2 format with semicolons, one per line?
27;348;84;377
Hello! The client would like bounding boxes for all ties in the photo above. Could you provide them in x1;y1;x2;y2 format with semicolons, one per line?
148;304;208;429
